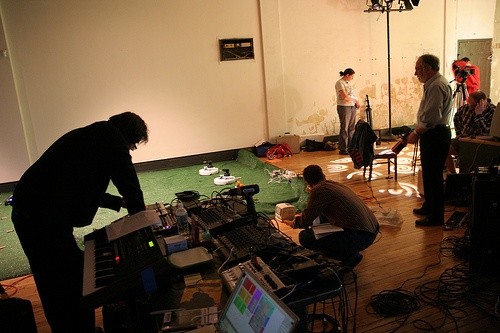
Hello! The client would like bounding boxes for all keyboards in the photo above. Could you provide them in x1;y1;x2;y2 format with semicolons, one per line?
479;136;493;139
445;211;468;227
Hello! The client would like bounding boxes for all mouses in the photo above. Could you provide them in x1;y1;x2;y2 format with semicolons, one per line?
444;225;453;230
470;136;476;139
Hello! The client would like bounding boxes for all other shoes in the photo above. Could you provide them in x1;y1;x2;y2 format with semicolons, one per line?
415;218;438;227
413;206;431;216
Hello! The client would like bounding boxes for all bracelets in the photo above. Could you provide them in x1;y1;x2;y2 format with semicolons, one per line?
413;129;420;136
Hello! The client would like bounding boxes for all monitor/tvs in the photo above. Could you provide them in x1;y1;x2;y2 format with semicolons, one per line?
489;102;500;139
445;173;487;207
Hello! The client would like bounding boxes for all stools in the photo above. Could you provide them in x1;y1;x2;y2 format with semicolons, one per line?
0;298;38;333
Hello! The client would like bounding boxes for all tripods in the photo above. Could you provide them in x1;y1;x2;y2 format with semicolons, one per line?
449;83;470;125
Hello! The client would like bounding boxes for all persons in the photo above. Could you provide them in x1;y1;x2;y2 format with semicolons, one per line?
461;57;481;105
454;91;496;172
282;166;381;274
335;68;361;155
11;111;148;333
406;55;453;225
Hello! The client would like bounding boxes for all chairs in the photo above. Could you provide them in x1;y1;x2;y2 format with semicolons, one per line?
348;119;397;181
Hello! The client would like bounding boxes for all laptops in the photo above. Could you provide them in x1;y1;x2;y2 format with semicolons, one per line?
185;269;300;333
